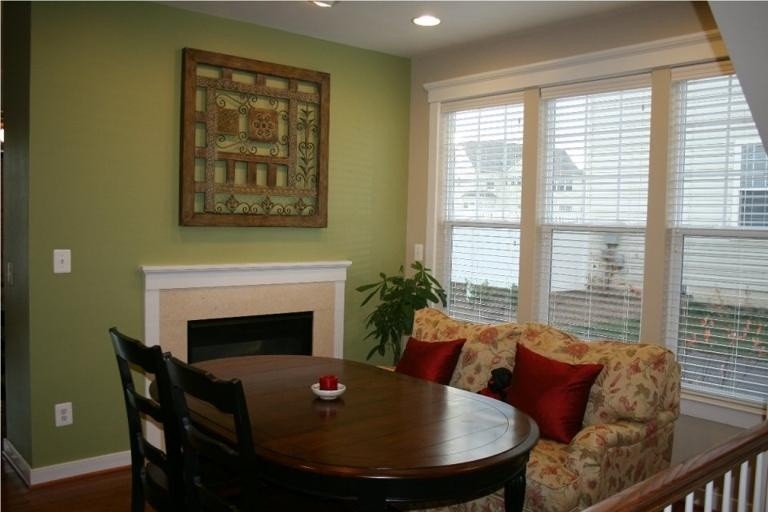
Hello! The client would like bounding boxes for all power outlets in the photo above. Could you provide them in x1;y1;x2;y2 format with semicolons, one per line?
54;401;74;427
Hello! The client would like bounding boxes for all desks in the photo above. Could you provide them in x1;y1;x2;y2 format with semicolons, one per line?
147;354;541;511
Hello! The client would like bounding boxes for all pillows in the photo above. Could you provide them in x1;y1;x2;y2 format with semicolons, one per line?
393;337;467;386
506;342;604;444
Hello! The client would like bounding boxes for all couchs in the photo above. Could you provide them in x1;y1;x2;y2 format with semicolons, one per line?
387;307;683;511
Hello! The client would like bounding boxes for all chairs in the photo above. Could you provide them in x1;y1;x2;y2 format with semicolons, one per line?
161;351;322;511
108;325;167;512
